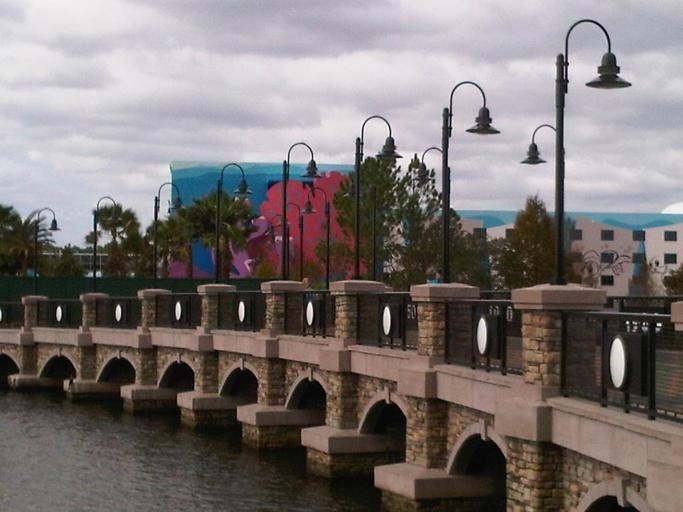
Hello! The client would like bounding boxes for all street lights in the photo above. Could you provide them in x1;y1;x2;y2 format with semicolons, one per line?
415;79;502;283
34;207;61;294
300;186;331;290
266;142;323;281
151;182;184;289
338;113;404;282
93;196;121;293
214;162;253;284
519;19;632;286
